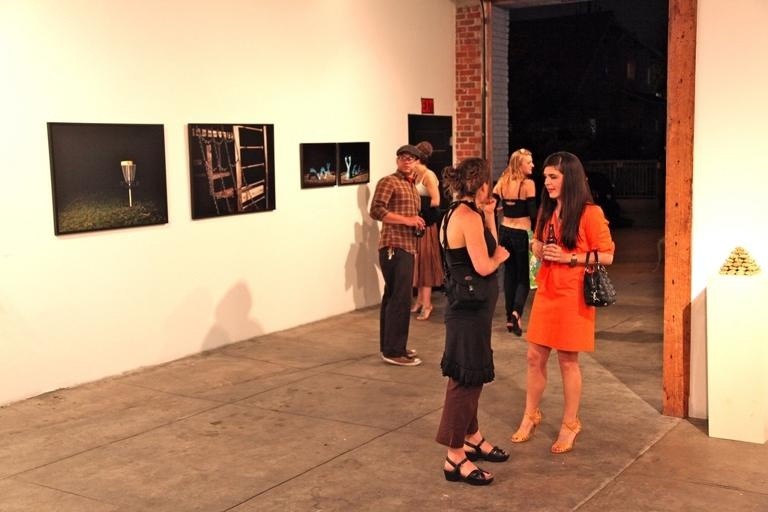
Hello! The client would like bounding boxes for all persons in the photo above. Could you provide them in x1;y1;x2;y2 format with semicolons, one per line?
511;151;615;454
492;148;538;337
370;145;422;367
411;141;441;320
434;156;511;484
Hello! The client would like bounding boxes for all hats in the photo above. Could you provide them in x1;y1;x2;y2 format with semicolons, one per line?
396;145;420;157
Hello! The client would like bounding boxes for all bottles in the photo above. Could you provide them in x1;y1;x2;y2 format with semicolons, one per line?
413;211;426;237
545;224;557;246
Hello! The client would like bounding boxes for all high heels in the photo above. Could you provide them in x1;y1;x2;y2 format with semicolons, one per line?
511;409;543;443
552;415;582;453
506;313;522;337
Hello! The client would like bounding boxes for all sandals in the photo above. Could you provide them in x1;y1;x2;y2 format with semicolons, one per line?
443;456;493;485
417;305;433;320
411;303;422;312
464;439;509;463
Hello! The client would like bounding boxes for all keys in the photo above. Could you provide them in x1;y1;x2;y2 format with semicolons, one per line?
387;247;395;261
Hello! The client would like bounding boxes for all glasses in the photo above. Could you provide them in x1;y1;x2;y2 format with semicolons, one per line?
400;155;413;161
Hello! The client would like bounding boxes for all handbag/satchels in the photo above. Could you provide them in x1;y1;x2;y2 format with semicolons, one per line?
584;266;616;306
442;262;488;310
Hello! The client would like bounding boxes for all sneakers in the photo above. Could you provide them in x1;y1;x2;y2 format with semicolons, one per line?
380;350;416;357
382;353;422;366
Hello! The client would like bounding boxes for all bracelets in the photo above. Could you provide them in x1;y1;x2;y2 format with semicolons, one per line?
571;254;577;268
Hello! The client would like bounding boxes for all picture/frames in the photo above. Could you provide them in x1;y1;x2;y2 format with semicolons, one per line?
187;123;276;220
337;142;371;186
300;142;337;189
46;121;169;236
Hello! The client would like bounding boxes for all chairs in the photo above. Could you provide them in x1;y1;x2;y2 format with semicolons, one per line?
652;216;664;272
